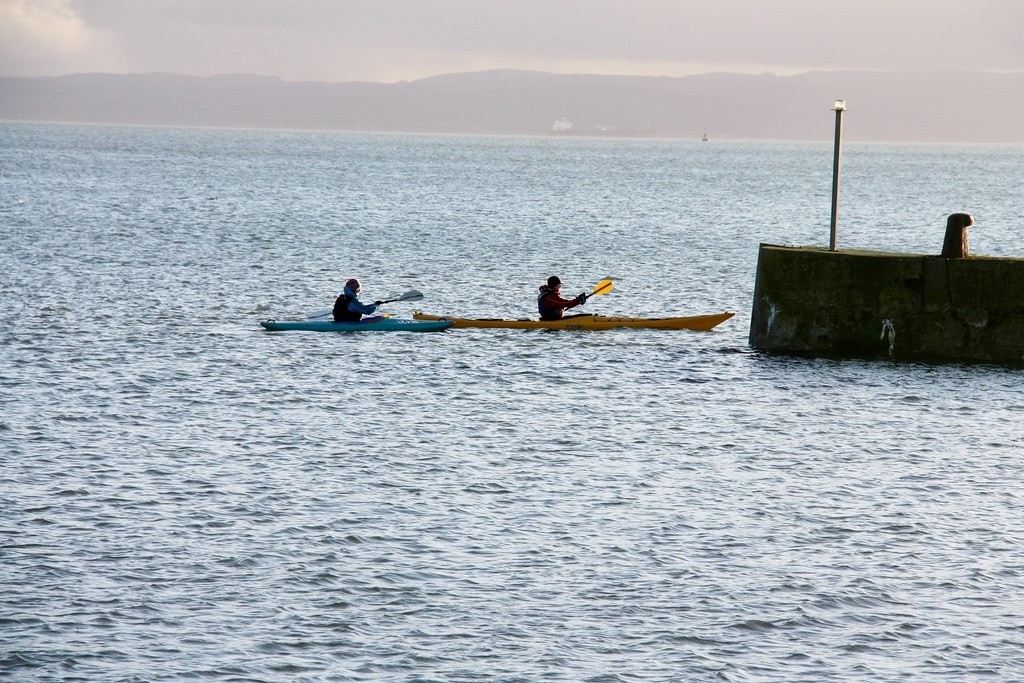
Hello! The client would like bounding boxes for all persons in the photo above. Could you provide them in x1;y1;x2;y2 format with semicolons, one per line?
333;279;382;322
538;276;586;321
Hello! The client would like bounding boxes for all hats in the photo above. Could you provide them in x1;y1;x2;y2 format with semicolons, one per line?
346;279;359;292
547;276;562;289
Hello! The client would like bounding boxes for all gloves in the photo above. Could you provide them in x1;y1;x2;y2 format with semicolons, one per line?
576;293;588;305
375;301;382;306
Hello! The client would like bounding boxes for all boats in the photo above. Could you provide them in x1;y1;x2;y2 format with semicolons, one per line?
260;315;456;331
413;312;736;332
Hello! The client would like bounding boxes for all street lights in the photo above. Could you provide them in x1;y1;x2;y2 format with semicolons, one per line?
829;101;847;251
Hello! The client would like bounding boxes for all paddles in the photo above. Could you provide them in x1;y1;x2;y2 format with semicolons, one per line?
565;279;613;312
308;291;424;321
